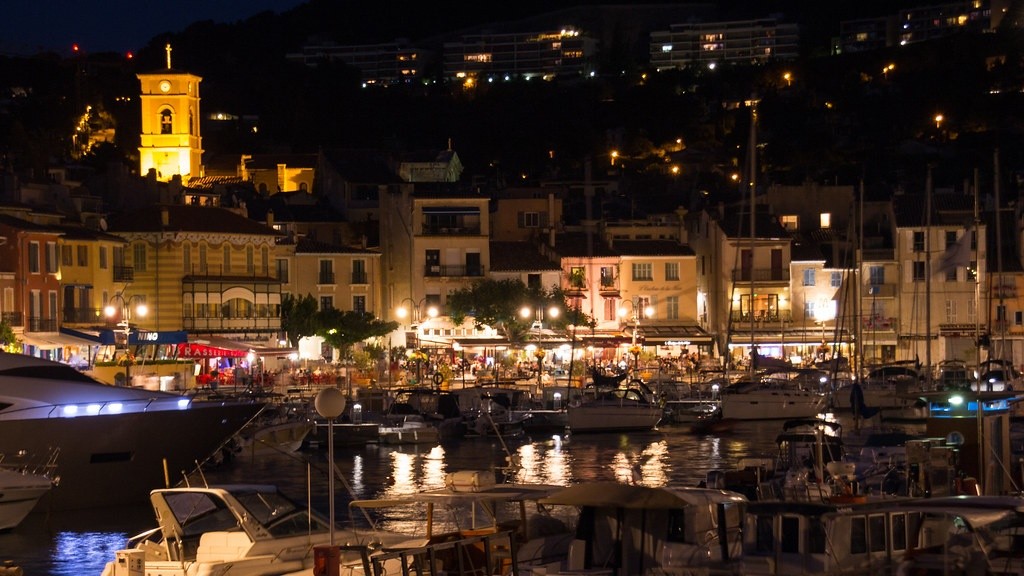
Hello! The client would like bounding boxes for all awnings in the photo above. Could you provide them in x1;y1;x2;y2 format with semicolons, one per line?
57;328;187;360
172;343;248;386
14;331;101;367
218;340;300;384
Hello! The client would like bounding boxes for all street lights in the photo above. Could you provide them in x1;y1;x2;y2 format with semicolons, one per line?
617;300;656;380
396;298;439;384
103;294;149;386
518;305;560;390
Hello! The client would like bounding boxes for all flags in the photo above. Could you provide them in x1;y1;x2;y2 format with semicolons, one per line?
932;228;970;274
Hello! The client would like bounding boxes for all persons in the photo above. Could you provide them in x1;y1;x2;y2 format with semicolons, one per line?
211;367;221;376
256;368;276;387
656;352;699;372
402;355;633;379
68;355;168;366
296;366;321;385
231;361;247;370
740;353;831;372
697;479;706;488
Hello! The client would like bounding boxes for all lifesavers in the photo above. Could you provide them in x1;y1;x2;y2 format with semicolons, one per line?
433;372;444;384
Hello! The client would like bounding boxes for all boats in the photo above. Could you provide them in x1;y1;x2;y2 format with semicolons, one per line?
0;441;62;534
0;347;271;516
93;87;1024;576
